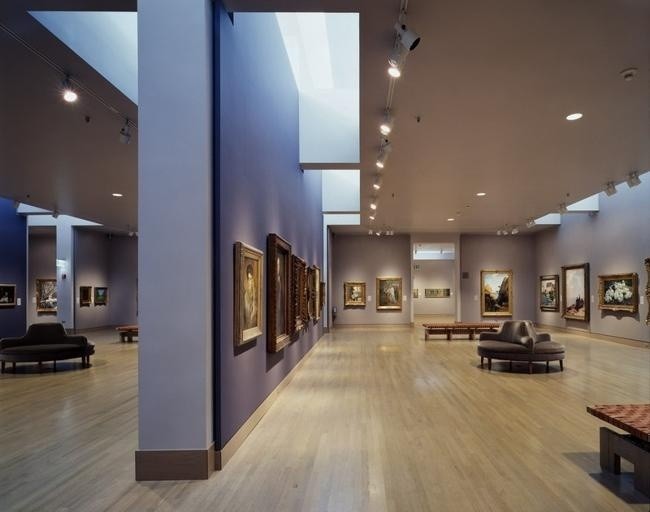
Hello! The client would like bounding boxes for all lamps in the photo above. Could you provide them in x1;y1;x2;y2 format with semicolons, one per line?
366;20;420;236
62;78;132;145
628;172;641;188
606;183;616;196
495;203;568;237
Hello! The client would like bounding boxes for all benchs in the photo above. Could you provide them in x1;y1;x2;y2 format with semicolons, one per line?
422;323;499;340
583;405;650;497
116;326;138;343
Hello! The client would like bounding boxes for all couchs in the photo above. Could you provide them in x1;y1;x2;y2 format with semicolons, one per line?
478;320;565;373
0;324;94;372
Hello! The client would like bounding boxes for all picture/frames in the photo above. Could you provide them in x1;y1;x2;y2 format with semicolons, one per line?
376;277;402;310
0;284;17;307
540;257;650;322
35;277;58;314
79;286;110;310
480;269;512;316
233;232;325;353
344;281;366;306
